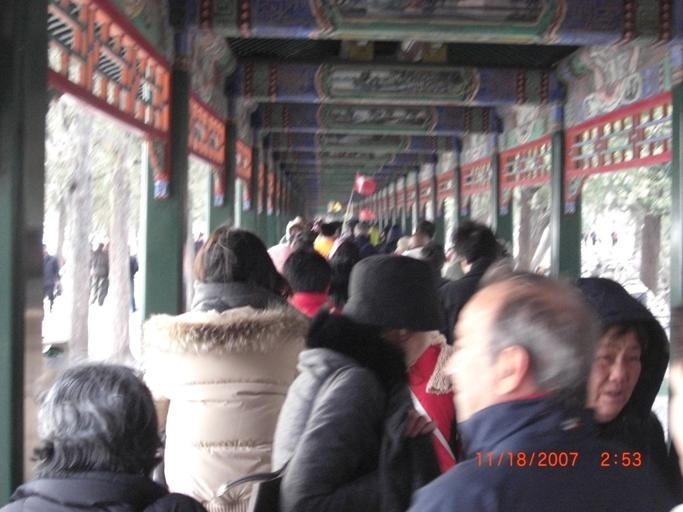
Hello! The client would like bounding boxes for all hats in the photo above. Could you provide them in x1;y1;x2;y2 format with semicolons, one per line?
342;254;448;333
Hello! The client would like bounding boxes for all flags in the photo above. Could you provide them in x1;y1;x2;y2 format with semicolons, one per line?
352;170;378;197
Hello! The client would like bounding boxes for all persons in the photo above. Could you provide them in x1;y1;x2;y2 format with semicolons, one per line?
143;216;683;511
129;255;138;313
42;241;62;311
89;243;107;307
0;362;209;511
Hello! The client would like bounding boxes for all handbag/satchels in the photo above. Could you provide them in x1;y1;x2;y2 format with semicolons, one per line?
218;359;440;510
